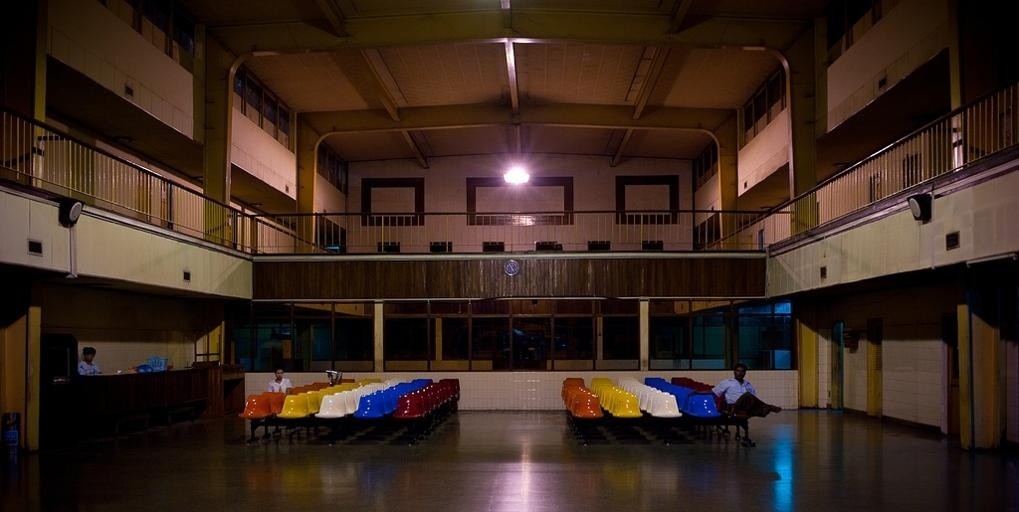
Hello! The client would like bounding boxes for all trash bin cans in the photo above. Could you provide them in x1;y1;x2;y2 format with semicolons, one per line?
4;412;20;446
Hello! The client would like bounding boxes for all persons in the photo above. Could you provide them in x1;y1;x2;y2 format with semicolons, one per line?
268;368;294;396
78;346;103;375
689;364;782;417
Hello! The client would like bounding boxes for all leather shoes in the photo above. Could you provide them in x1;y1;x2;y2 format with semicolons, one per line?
769;405;781;413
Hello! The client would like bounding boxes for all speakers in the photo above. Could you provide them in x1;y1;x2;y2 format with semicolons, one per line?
906;193;932;221
58;197;84;227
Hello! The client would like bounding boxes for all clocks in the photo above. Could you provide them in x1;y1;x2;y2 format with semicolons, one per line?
504;259;520;278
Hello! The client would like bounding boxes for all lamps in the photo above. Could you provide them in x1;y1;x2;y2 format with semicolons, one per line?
503;124;530;185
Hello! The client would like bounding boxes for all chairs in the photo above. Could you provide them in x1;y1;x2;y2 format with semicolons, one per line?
236;378;459;449
560;375;752;446
